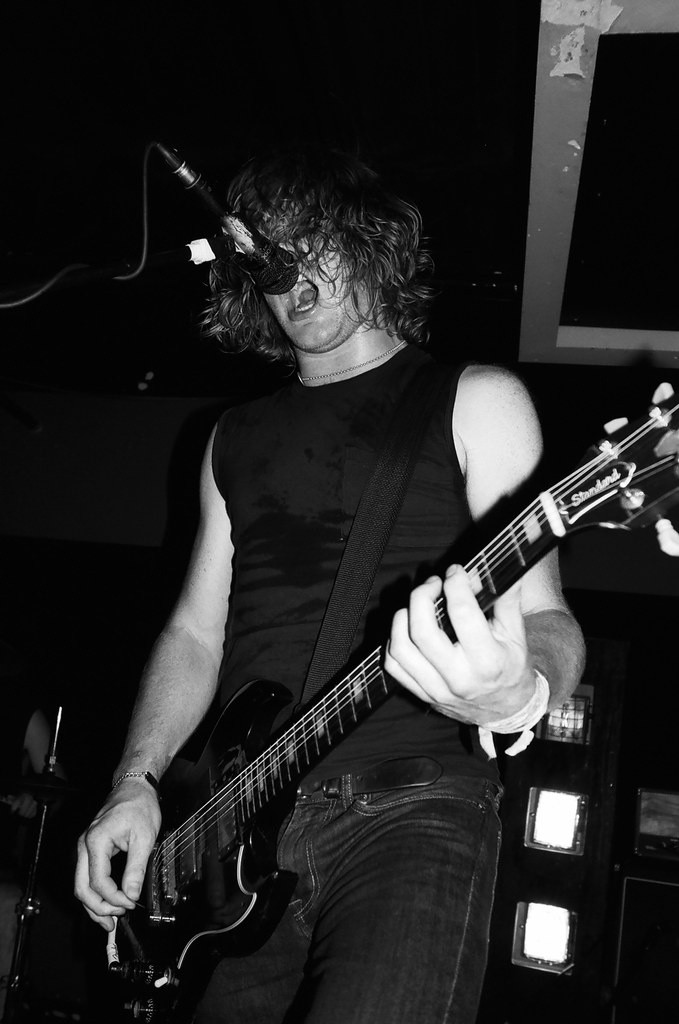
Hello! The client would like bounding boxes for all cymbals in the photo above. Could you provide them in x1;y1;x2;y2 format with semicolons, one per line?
1;769;87;801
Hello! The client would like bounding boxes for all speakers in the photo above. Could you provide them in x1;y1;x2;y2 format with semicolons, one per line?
598;863;679;1024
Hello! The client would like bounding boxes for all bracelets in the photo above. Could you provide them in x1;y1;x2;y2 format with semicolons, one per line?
477;667;552;761
111;770;161;793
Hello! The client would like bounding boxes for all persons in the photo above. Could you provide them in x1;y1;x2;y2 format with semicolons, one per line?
70;135;590;1024
0;641;65;1024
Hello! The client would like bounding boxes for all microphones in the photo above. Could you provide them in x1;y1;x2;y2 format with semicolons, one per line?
152;142;298;296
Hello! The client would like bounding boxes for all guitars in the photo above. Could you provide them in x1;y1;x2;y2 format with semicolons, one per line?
24;380;679;1023
